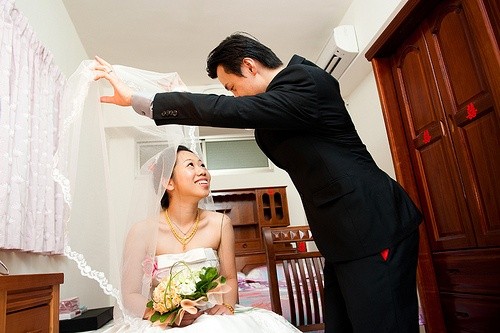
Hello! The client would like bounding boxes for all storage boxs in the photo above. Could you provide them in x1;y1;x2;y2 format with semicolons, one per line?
59;296;83;320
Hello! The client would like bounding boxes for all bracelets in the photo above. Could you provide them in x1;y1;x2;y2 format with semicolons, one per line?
222;303;235;312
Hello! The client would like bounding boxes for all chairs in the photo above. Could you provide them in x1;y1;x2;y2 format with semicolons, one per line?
262;227;329;333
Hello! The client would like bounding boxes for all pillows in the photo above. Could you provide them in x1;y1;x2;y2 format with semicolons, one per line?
246;265;319;287
236;272;245;283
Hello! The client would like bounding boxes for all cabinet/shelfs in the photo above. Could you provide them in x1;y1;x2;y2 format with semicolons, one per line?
365;1;499;332
203;185;291;275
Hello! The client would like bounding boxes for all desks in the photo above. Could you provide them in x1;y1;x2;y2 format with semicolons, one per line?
0;269;66;333
59;306;115;333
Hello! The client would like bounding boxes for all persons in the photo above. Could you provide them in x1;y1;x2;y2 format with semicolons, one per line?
116;145;303;333
90;32;424;333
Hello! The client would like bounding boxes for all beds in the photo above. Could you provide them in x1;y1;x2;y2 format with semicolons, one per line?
234;253;425;333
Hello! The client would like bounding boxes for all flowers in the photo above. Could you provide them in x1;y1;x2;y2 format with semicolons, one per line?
140;260;229;326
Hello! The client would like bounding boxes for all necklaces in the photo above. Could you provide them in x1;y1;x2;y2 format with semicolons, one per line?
164;207;201;251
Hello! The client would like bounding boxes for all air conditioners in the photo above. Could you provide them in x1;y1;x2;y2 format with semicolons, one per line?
315;25;360;78
